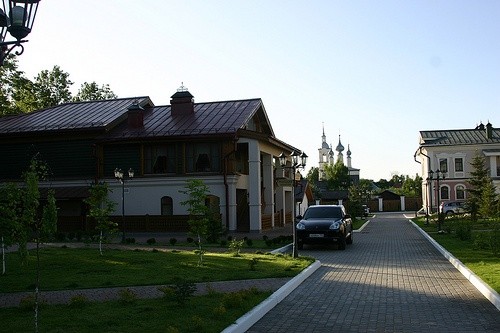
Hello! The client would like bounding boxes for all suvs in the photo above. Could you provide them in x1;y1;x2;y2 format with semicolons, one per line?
295;204;353;249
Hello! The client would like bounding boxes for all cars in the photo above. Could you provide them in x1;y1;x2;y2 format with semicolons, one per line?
361;204;370;217
439;200;472;216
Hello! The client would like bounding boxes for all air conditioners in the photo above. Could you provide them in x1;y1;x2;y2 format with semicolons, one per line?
275;167;285;179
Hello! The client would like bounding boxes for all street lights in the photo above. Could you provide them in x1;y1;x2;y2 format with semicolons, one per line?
418;177;435;226
428;170;434;213
276;151;309;258
357;189;367;219
429;169;446;233
113;168;136;242
